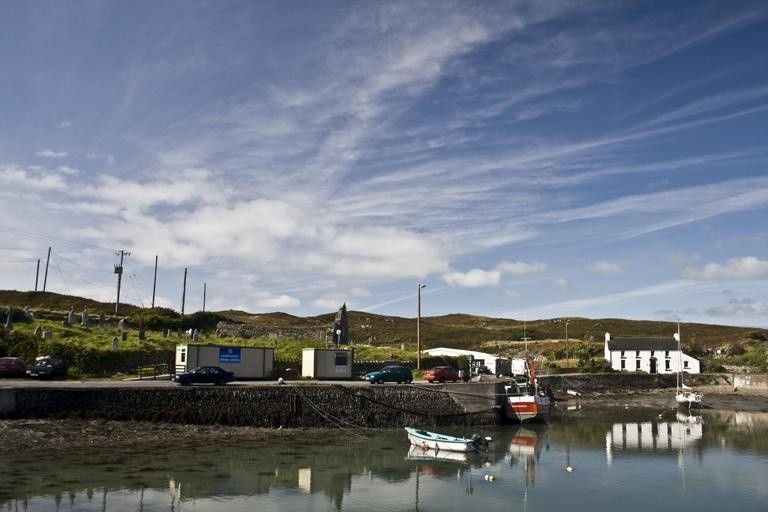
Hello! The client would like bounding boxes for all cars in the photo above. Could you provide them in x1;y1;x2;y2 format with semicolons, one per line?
0;357;27;378
172;365;235;386
25;359;56;381
422;366;456;384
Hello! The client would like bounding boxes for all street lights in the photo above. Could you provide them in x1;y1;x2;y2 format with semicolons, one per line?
565;319;571;373
417;283;426;372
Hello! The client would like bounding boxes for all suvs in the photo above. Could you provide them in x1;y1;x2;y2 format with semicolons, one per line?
364;365;413;385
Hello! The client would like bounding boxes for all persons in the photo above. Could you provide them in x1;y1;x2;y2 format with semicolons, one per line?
193;328;198;334
216;327;221;336
139;318;145;333
35;325;41;337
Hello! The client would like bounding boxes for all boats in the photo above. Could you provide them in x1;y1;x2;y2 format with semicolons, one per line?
403;426;492;452
504;358;555;426
674;391;702;404
